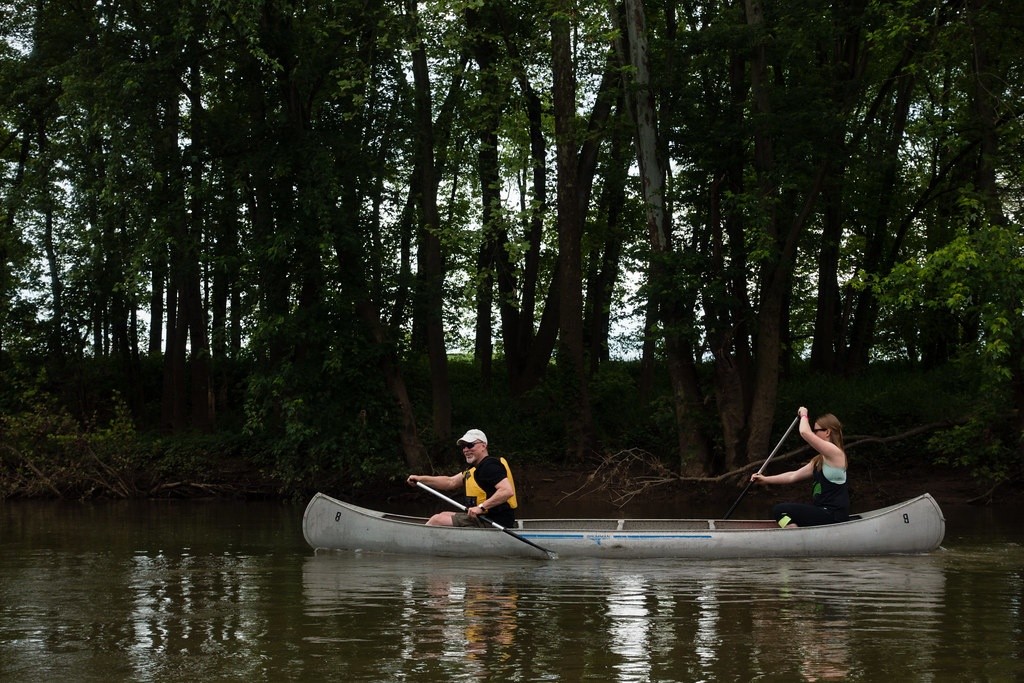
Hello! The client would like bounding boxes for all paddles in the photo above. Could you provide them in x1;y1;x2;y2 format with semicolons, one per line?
722;413;800;517
409;477;562;561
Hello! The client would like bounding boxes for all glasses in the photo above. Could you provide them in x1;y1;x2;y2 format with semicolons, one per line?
814;429;828;434
461;442;483;449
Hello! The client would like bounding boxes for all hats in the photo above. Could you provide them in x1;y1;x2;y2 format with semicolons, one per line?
457;428;488;446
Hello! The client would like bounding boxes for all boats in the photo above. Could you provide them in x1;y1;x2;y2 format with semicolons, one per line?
301;492;946;562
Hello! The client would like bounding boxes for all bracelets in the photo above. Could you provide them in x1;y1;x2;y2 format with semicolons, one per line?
801;415;807;419
478;504;487;512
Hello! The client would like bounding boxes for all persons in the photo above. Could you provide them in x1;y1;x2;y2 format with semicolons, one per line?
407;428;518;528
750;406;850;528
